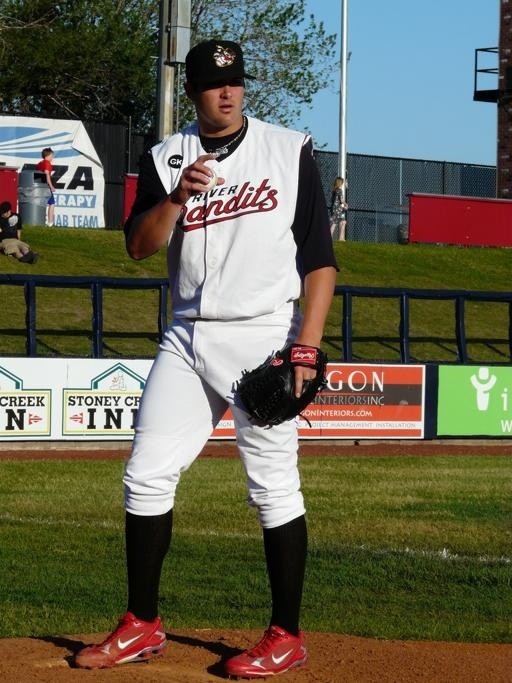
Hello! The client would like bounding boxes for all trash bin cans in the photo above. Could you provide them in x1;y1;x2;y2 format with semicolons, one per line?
0;167;51;225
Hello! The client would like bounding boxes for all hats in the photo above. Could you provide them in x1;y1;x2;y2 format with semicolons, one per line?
184;38;256;86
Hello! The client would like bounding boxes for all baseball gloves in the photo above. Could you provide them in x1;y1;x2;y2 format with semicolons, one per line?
233;342;325;426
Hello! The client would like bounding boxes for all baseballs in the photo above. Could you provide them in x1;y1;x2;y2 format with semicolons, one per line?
199;167;216;191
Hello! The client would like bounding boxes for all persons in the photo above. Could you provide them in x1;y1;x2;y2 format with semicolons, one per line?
325;176;350;242
0;200;40;264
36;146;58;229
71;39;342;680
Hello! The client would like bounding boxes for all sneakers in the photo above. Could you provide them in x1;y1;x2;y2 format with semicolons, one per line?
224;625;308;680
74;611;169;670
19;251;39;263
